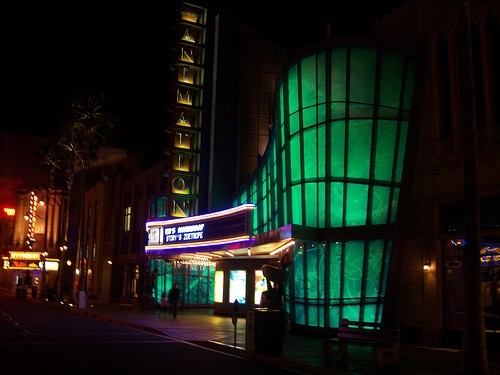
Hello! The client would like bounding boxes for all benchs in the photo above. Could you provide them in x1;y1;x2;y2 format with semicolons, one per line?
320;319;403;372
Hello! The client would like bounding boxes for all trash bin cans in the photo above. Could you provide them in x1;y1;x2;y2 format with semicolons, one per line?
16;288;28;302
245;307;288;358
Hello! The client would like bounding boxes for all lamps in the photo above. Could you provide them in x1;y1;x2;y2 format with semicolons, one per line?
422;254;432;271
